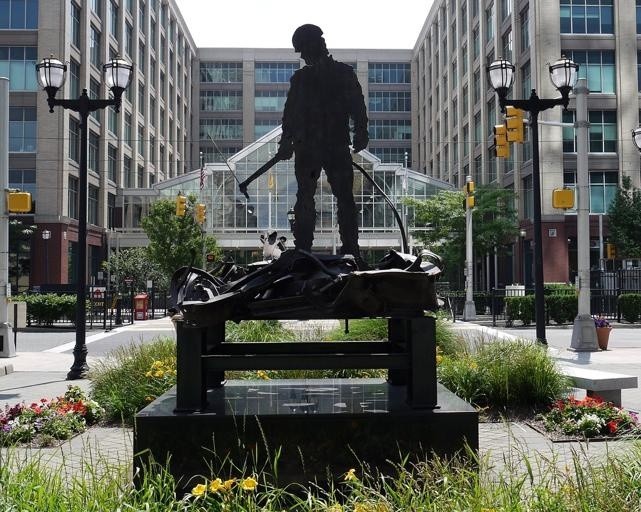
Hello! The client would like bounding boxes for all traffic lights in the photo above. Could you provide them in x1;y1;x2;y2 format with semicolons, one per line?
175;195;186;216
462;185;466;194
492;122;510;161
466;181;474;193
467;195;475;209
195;203;206;222
503;105;525;145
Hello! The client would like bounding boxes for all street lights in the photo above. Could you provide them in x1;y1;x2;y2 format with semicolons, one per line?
32;51;139;381
287;206;318;233
629;125;641;183
484;51;581;349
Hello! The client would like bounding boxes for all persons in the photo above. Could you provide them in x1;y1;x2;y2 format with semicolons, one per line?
274;23;369;274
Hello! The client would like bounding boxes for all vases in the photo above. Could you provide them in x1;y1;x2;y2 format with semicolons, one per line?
597;327;614;351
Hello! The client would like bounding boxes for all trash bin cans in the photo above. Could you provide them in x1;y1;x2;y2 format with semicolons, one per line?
134;293;148;321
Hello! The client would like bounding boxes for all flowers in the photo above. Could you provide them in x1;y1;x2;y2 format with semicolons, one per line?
594;314;614;326
